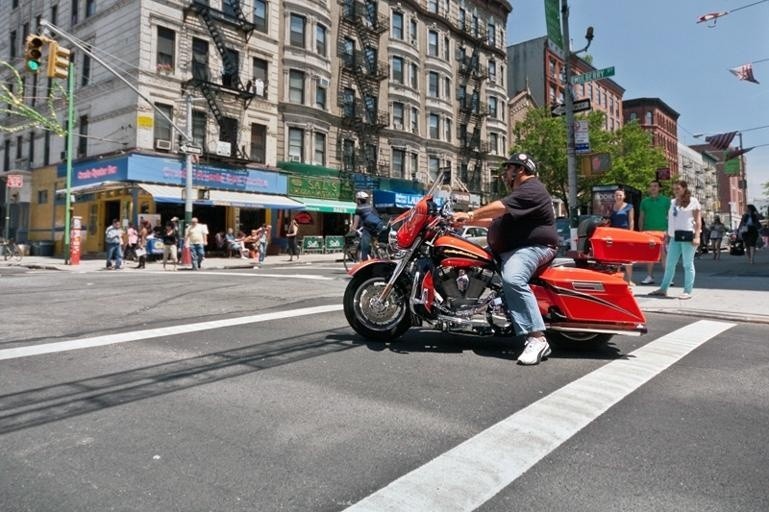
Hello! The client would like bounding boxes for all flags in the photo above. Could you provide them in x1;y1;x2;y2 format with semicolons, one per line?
698;11;728;28
730;63;760;85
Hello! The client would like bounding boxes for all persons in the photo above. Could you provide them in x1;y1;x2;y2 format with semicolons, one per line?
710;204;769;260
602;188;636;286
351;192;373;260
286;219;301;261
648;180;701;299
215;224;272;270
452;153;560;365
638;180;674;286
104;216;211;270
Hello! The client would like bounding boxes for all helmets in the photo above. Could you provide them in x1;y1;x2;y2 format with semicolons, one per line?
502;153;537;173
356;192;369;200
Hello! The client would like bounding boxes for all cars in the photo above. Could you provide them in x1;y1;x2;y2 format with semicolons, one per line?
554;214;610;257
455;225;489;250
354;224;397;246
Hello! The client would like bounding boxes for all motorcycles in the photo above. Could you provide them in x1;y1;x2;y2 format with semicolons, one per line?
342;170;668;354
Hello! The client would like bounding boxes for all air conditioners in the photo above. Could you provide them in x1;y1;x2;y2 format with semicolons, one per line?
155;139;171;151
291;155;300;163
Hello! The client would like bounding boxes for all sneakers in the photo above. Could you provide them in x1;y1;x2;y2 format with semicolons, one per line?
678;293;692;299
640;274;655;284
516;336;551;364
648;289;666;295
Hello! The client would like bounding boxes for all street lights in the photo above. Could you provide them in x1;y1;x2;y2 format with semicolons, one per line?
693;131;746;214
561;0;595;253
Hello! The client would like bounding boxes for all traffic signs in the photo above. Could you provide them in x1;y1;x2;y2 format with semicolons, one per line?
177;143;203;156
550;98;591;117
7;173;24;189
544;0;563;49
570;65;615;85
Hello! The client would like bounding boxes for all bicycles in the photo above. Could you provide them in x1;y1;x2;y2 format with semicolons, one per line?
0;236;23;262
344;216;392;274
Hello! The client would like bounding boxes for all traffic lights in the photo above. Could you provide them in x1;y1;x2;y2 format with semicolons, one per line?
23;33;44;75
580;150;612;178
48;40;70;79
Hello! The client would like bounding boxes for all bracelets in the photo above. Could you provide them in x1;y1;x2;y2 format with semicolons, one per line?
468;211;474;223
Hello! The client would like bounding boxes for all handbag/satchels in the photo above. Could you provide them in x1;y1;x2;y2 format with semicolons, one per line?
730;241;744;255
362;213;384;233
675;231;693;241
739;226;747;234
